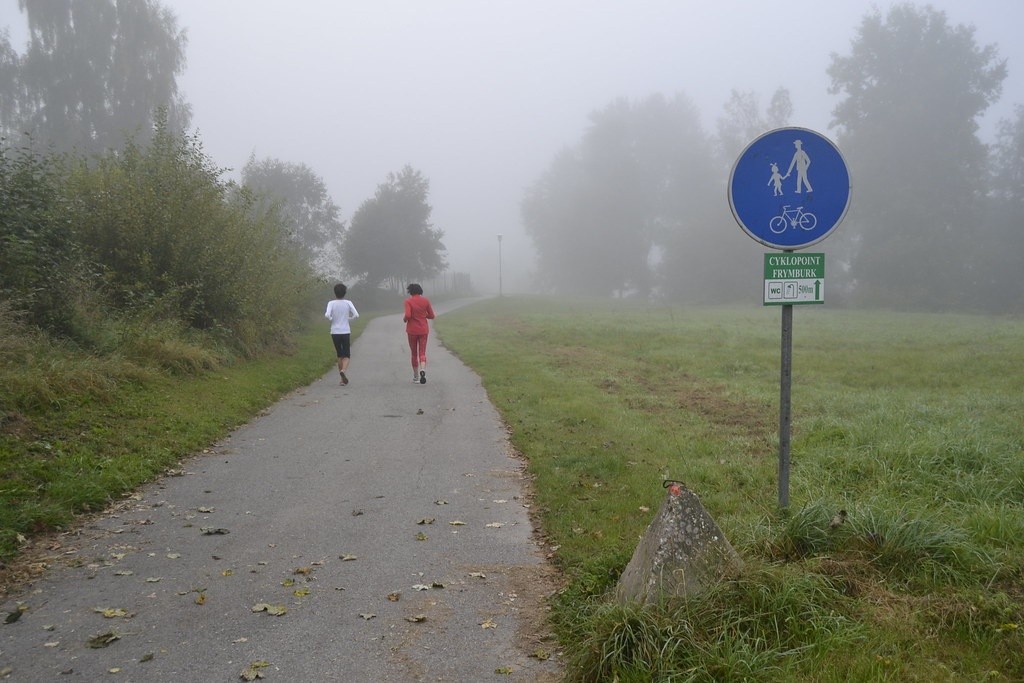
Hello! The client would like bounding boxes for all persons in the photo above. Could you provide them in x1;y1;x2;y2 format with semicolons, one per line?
403;284;434;384
325;284;359;386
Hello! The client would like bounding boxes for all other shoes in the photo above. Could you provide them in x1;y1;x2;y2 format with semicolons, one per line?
420;370;427;386
340;379;345;386
413;376;420;384
340;371;349;385
